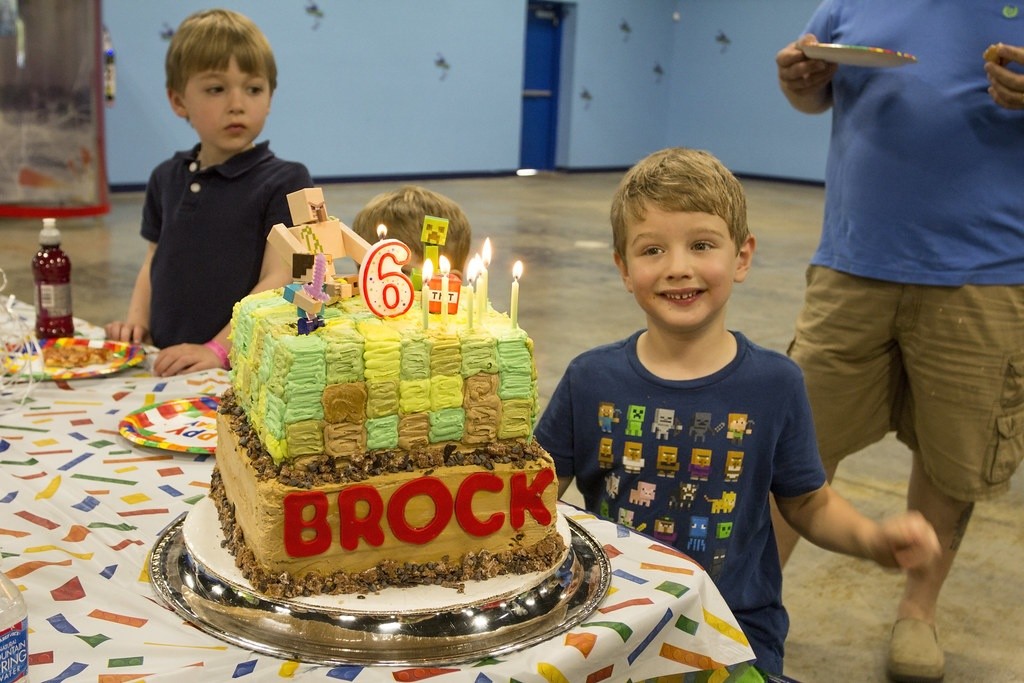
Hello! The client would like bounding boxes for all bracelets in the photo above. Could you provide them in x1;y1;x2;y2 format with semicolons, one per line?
204;340;231;370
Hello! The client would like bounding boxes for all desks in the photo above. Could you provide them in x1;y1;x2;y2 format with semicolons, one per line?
0;293;759;683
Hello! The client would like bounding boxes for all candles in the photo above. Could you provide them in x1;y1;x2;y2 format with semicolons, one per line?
480;262;489;315
422;280;430;329
358;231;414;319
511;276;519;329
476;273;484;323
467;279;473;327
440;273;449;326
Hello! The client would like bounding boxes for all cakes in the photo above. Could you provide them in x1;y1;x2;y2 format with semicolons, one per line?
208;267;565;601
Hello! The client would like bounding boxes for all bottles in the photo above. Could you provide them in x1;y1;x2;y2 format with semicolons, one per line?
0;546;30;683
31;218;75;341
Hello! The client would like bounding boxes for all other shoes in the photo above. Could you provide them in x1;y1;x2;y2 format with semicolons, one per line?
887;613;945;683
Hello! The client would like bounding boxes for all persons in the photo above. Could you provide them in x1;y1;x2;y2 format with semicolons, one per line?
765;0;1024;682
534;148;943;683
104;10;315;377
353;185;473;273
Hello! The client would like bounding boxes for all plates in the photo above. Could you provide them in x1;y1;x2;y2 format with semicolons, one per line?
795;42;919;70
0;337;146;381
119;396;221;454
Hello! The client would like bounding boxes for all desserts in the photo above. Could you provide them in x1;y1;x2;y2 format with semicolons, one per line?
45;348;113;368
